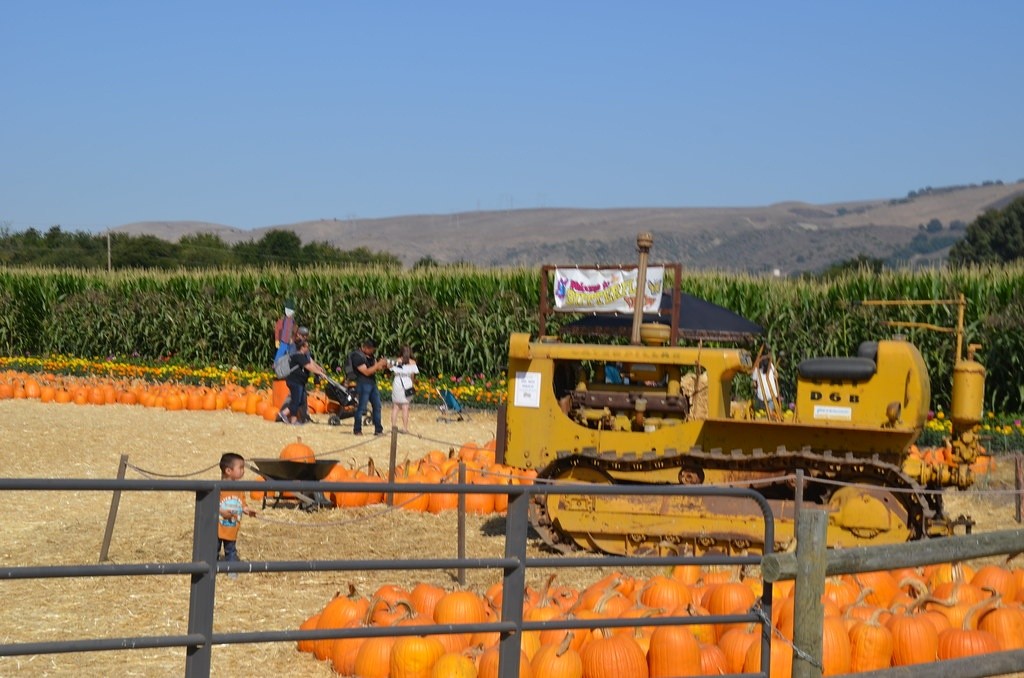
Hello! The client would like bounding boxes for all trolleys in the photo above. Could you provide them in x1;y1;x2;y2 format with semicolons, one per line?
242;458;341;515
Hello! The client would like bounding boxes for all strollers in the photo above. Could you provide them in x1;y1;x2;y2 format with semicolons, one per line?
320;373;374;426
435;387;473;423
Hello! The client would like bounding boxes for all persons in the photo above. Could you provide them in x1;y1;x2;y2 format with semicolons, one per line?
278;337;327;425
387;345;419;433
350;340;387;435
217;453;249;579
750;351;778;411
276;327;324;422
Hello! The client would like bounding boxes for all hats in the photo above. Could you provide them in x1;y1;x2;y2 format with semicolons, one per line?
298;327;309;336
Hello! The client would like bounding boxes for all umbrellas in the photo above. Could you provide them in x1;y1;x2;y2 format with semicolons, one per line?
560;287;763;346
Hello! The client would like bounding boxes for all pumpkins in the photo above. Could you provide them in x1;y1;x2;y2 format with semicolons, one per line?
824;559;1024;678
0;368;352;423
910;444;997;474
298;564;794;678
250;436;539;513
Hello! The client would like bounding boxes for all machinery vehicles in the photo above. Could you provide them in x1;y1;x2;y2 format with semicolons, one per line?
495;230;987;563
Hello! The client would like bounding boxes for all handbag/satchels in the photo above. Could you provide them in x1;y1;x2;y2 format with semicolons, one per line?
405;387;416;397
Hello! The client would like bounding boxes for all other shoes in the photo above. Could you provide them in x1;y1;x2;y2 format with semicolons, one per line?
279;412;289;424
375;431;384;436
295;420;302;425
354;431;363;436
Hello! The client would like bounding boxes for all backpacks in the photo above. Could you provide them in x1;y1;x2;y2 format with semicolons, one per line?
275;351;303;379
